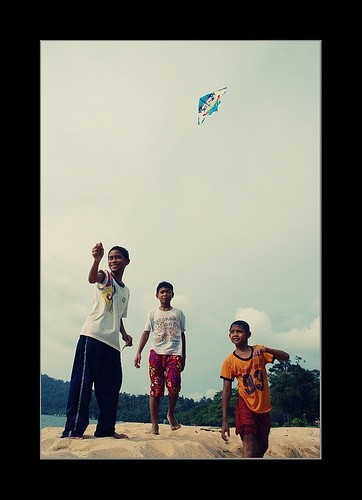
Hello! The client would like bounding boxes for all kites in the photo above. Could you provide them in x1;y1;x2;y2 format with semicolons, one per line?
196;86;228;125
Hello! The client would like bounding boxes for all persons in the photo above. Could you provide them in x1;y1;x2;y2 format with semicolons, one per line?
221;320;289;458
134;281;186;435
60;242;133;439
